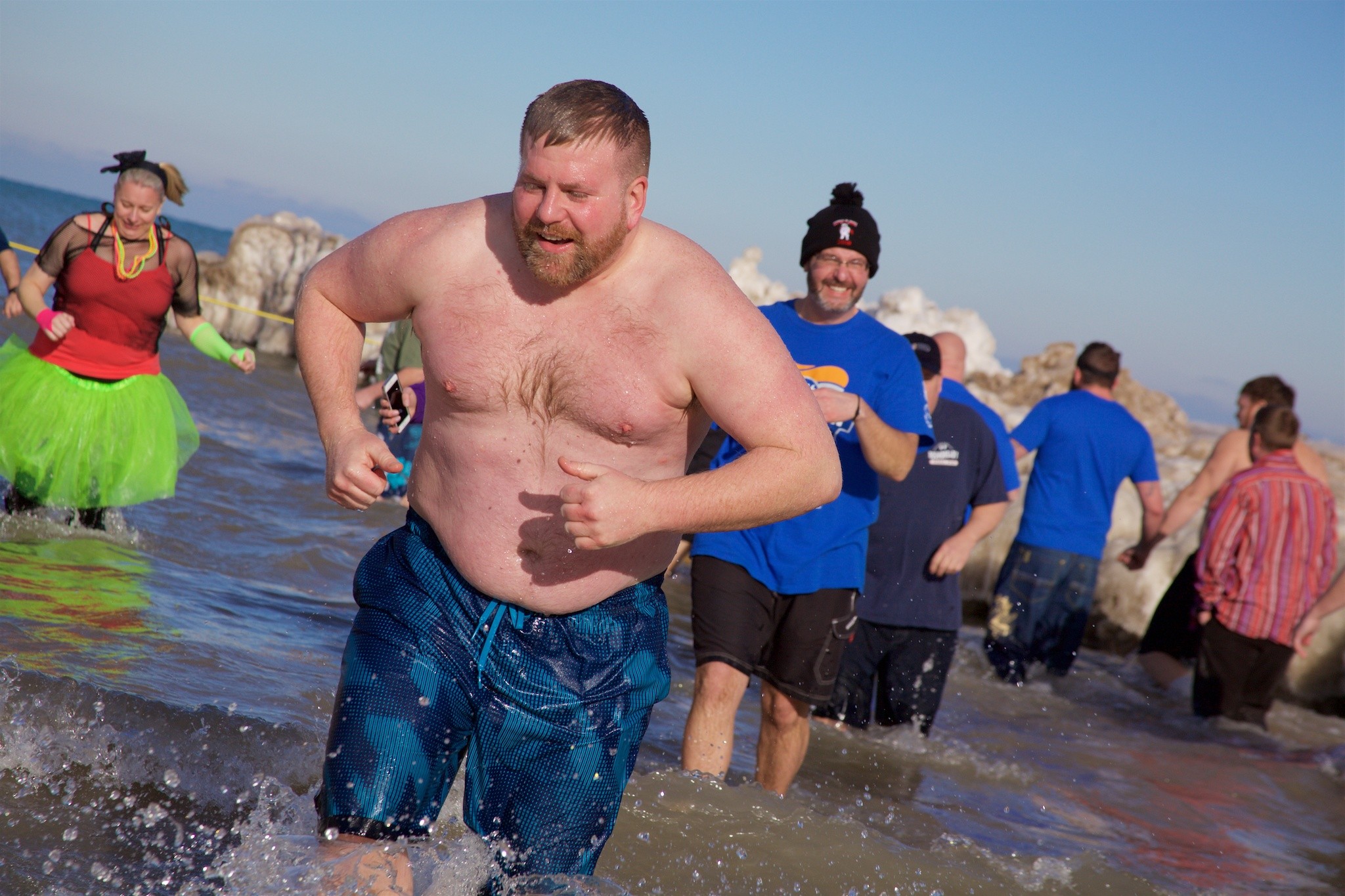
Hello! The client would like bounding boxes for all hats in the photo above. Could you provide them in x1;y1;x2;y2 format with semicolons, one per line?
801;182;881;279
902;332;941;375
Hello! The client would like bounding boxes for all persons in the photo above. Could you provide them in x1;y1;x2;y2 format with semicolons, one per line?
676;181;937;804
381;379;427;435
0;149;254;530
927;333;1021;531
811;329;1011;738
1135;374;1326;697
293;81;845;896
658;427;723;576
0;225;24;321
1290;571;1345;658
973;344;1168;687
1191;404;1341;729
